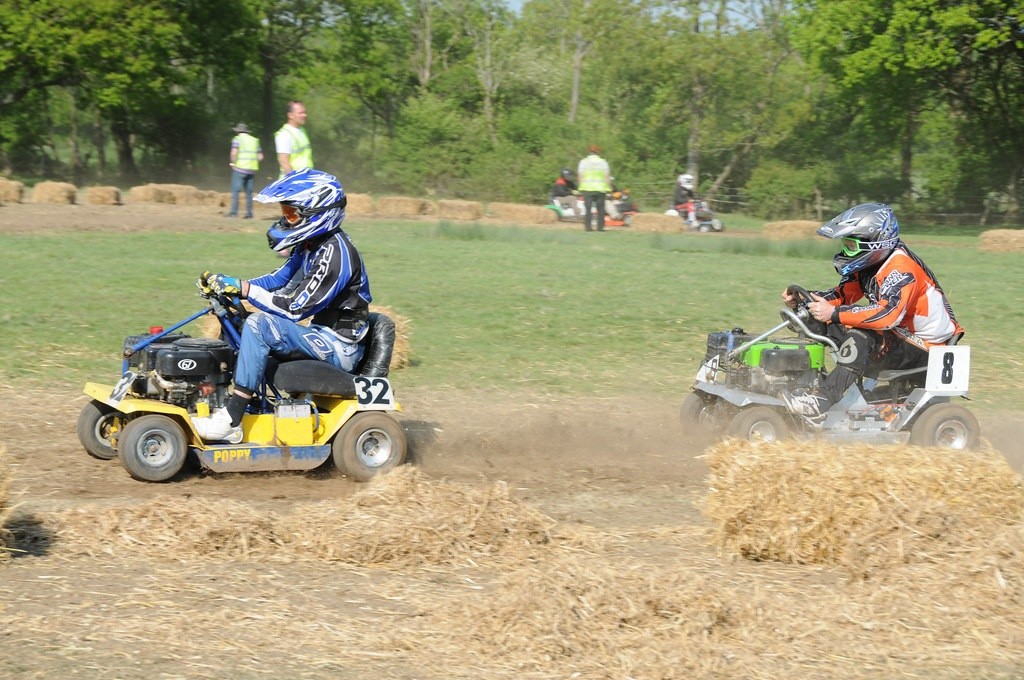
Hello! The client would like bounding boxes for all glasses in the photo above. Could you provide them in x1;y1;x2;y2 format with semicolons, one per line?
278;202;304;226
840;237;862;257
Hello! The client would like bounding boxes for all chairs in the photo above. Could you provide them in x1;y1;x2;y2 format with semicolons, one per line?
267;311;397;398
860;328;963;412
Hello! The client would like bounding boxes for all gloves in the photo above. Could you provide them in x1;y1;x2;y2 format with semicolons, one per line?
206;273;243;297
196;271;216;298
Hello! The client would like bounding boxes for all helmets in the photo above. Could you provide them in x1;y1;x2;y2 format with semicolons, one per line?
252;168;346;252
678;175;694;190
816;202;899;276
560;168;572;179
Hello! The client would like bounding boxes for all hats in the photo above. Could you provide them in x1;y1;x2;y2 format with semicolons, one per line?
232;123;252;133
591;145;601;152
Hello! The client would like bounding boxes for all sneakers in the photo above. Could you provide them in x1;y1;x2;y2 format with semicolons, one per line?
191;406;244;444
783;389;828;429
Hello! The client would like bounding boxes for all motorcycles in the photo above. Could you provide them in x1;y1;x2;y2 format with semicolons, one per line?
667;201;723;232
679;284;981;460
80;270;407;486
547;190;634;229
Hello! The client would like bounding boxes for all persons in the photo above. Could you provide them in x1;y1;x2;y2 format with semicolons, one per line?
674;174;708;227
273;100;314;258
191;168;373;445
223;122;264;219
552;144;630;232
781;203;964;431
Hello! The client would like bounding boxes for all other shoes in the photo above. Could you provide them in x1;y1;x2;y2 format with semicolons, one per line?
225;213;237;218
243;216;253;220
